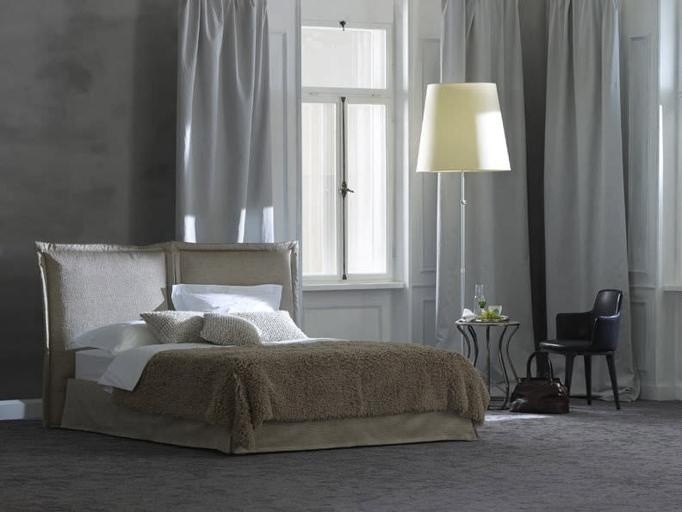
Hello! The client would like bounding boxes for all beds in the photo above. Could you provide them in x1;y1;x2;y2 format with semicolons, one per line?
31;238;488;454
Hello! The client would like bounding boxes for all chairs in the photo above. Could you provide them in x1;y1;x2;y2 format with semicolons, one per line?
528;289;623;408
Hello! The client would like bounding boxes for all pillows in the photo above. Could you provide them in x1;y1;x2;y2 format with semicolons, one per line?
196;313;263;347
241;312;310;344
168;283;284;317
136;308;204;343
66;320;157;348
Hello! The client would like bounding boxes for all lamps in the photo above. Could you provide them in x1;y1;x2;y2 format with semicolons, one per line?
415;82;512;360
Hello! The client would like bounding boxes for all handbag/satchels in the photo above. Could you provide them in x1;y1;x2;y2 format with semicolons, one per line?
509;377;570;414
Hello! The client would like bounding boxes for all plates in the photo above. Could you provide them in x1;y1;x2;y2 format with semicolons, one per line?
499;315;508;320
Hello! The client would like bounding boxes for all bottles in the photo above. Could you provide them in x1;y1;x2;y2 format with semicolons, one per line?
471;283;486;321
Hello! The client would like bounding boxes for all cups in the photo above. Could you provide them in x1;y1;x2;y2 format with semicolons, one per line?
488;305;503;316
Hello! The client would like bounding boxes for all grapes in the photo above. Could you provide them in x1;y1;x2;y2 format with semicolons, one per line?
479;311;500;319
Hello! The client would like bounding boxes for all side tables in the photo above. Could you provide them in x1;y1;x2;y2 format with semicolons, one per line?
452;319;520;415
